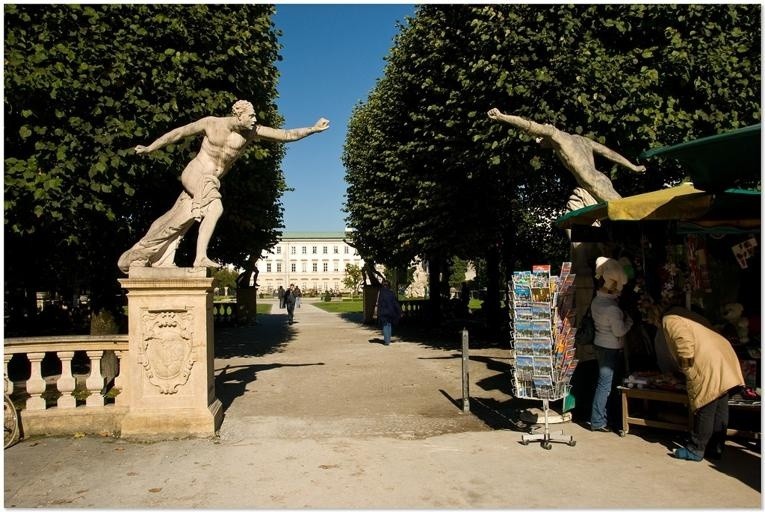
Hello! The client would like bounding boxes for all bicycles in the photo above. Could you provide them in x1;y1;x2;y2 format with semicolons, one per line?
4;393;19;449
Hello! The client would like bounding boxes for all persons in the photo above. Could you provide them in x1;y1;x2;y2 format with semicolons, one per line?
117;99;331;272
277;284;301;325
648;298;746;462
589;257;634;433
373;280;403;346
486;106;647;217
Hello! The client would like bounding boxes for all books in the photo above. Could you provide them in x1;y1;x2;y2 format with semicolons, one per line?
512;262;580;400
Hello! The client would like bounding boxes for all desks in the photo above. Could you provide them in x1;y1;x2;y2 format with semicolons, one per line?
620;388;692;437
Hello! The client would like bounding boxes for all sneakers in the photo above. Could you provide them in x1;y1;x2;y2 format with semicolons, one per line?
596;426;612;431
673;448;703;461
